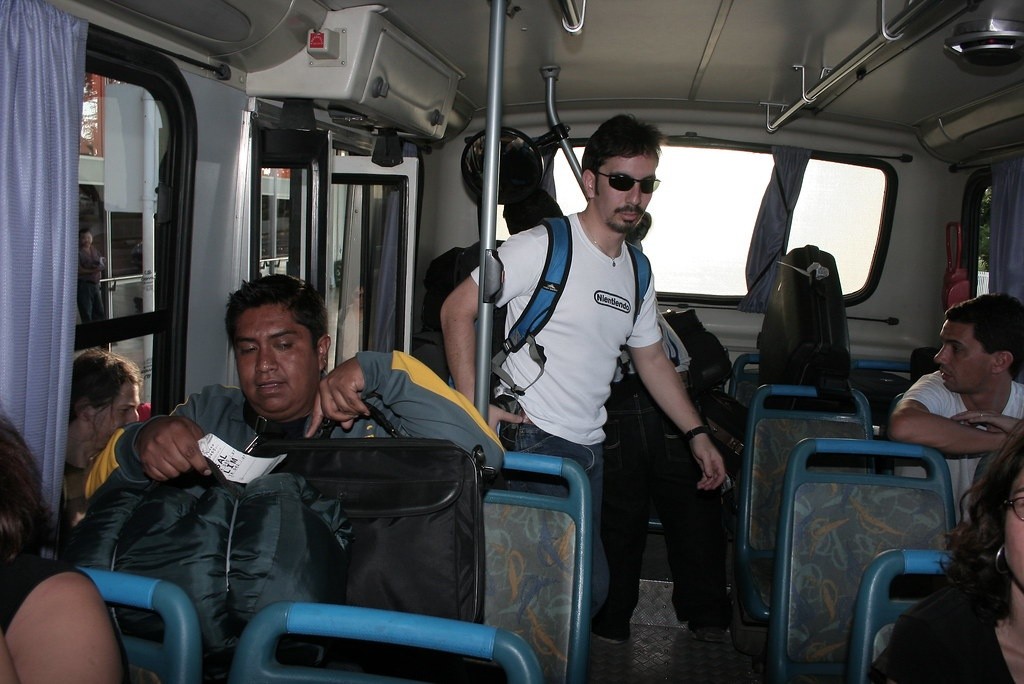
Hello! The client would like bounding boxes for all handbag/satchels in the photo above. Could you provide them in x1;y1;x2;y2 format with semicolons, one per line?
258;401;491;628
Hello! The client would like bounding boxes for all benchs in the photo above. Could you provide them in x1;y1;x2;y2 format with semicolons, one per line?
729;353;911;428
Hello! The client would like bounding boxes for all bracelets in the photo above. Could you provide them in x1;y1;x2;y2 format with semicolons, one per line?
680;425;712;443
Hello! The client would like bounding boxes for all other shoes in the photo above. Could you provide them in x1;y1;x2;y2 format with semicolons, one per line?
690;620;728;644
590;588;636;644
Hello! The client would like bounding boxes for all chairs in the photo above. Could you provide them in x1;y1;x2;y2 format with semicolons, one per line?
770;437;956;684
485;449;592;684
848;547;987;684
69;566;206;684
230;600;544;684
738;384;876;623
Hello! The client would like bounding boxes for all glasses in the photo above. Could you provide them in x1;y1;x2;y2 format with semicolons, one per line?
589;170;660;194
1001;496;1024;521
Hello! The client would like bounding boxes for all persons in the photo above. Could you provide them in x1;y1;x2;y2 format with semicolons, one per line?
341;285;369;364
58;346;140;548
0;414;119;684
82;274;505;684
440;113;734;644
868;424;1024;684
77;228;118;349
867;292;1024;550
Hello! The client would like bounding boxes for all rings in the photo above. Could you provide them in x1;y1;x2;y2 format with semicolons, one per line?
980;413;983;417
